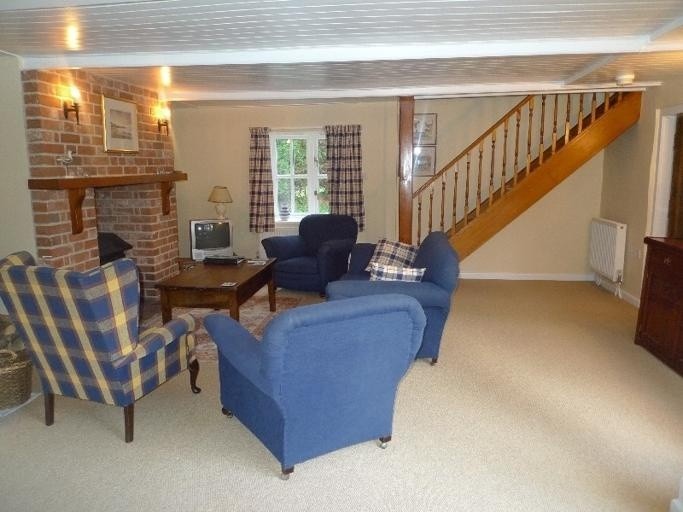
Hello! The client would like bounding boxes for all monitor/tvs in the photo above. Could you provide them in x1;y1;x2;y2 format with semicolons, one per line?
191;220;233;261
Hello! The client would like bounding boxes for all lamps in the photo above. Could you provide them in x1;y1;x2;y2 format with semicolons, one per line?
160;66;171;84
157;104;170;136
616;73;636;85
64;85;80;123
208;186;234;219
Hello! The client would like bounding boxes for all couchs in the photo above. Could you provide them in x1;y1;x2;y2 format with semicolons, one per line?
261;214;358;298
326;232;459;366
0;251;202;443
203;294;426;480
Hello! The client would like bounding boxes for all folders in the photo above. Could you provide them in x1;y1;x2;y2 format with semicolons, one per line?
202;255;247;266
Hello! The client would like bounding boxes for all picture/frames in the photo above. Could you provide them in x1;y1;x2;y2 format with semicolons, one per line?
413;146;436;176
101;94;138;153
412;113;437;144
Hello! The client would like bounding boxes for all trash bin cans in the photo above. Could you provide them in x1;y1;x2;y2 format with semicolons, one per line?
0;348;33;411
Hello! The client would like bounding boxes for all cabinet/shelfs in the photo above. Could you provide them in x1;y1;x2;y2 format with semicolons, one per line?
634;237;683;375
179;258;195;270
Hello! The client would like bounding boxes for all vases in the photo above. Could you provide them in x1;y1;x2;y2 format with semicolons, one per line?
279;204;290;221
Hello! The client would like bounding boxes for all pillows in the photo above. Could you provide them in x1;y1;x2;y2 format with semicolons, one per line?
365;238;420;272
370;263;427;283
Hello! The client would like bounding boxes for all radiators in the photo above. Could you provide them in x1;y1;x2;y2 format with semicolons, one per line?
589;219;627;298
